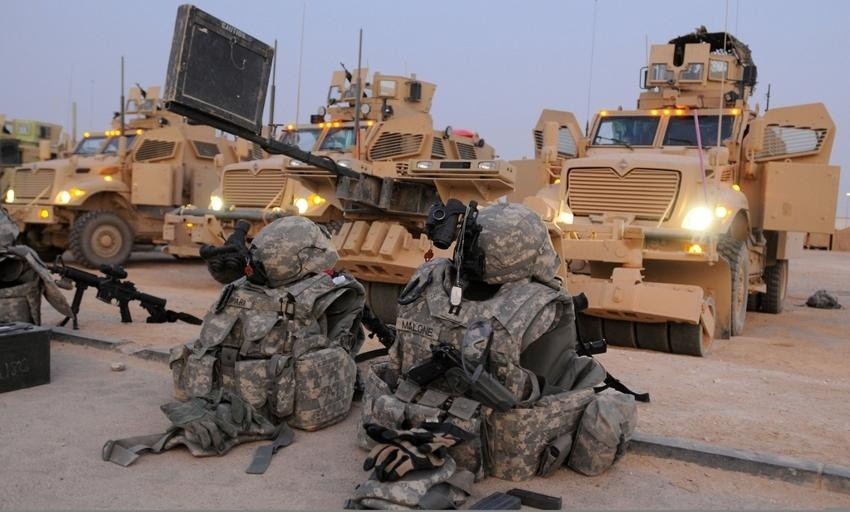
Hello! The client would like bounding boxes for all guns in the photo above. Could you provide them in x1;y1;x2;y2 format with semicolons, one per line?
136;83;162;112
361;305;395;348
339;64;367;98
572;291;607;355
49;264;202;330
408;342;518;413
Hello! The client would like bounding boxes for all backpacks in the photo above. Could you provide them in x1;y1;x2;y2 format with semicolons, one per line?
0;204;73;325
357;257;608;483
170;272;366;430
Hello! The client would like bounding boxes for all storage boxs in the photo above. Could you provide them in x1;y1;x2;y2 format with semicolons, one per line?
0;322;51;393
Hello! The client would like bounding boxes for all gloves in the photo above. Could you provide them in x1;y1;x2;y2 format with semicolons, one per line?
362;421;449;482
160;390;252;450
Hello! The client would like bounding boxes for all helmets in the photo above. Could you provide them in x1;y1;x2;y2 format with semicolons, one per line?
246;216;340;288
455;202;562;284
